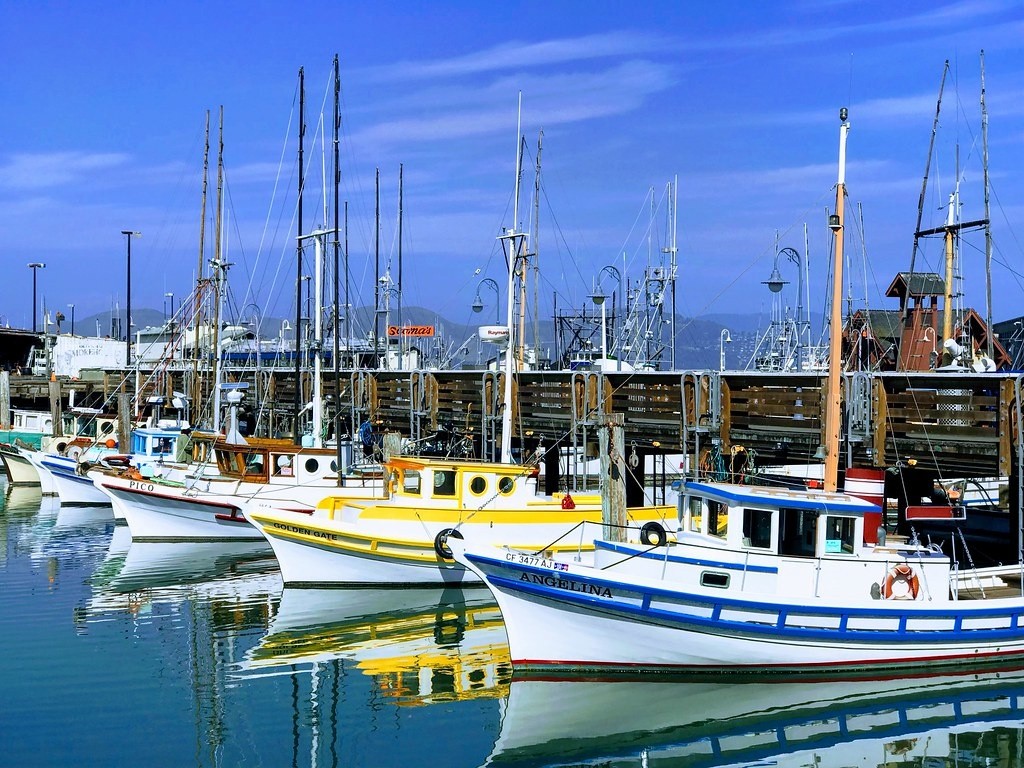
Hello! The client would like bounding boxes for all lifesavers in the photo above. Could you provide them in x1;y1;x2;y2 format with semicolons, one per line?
881;563;919;600
433;527;464;560
884;738;918;756
640;521;667;546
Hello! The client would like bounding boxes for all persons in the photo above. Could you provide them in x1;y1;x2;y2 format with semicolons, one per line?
176;429;195;464
237;407;247;431
975;351;983;361
937;353;942;367
358;416;376;459
517;448;536;464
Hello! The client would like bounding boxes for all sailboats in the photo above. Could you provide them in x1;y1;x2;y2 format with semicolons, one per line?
0;47;1024;688
